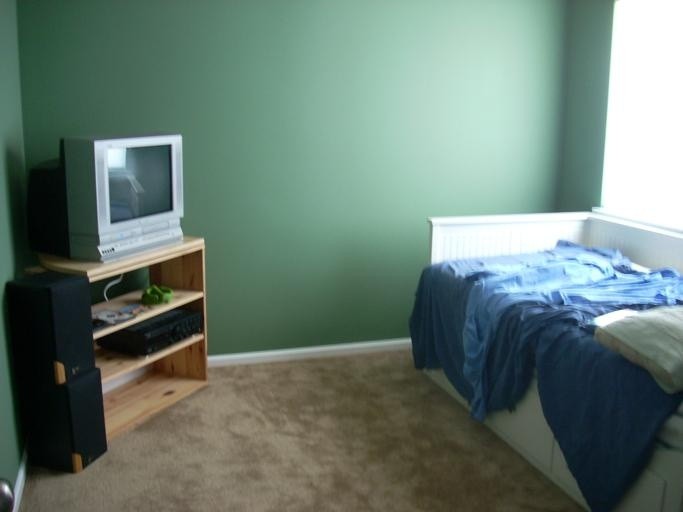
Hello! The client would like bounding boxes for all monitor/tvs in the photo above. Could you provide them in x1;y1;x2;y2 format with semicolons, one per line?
27;133;183;263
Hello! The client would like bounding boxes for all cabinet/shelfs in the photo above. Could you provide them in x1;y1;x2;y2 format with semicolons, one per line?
22;235;209;444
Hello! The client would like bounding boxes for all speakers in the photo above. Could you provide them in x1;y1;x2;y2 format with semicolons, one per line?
15;367;107;474
3;271;96;384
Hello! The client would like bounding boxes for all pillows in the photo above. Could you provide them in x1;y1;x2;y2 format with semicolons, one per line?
592;304;683;396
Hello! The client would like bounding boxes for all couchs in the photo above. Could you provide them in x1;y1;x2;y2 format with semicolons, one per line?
409;210;683;512
